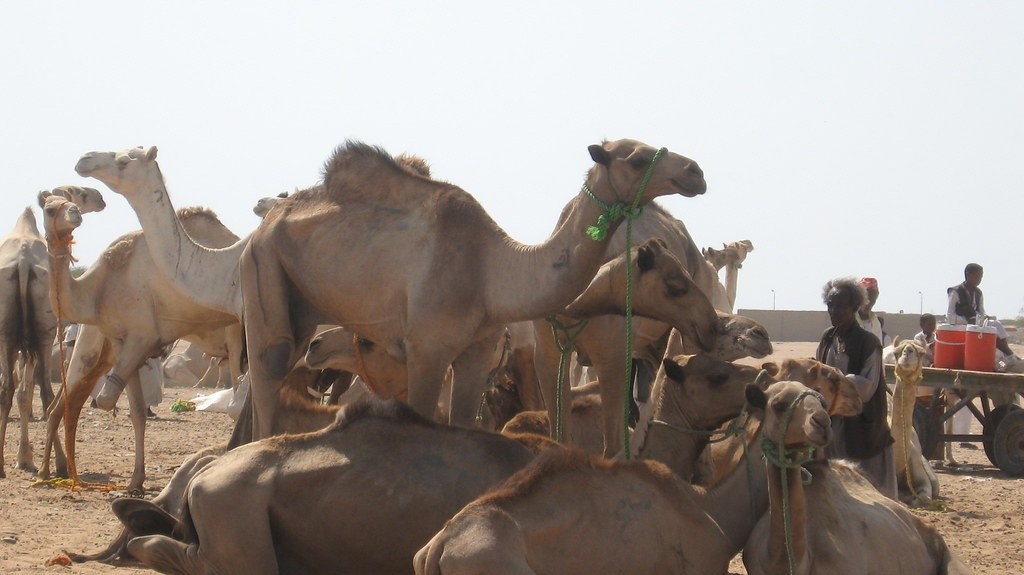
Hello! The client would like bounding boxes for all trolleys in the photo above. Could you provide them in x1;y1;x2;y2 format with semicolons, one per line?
885;363;1024;477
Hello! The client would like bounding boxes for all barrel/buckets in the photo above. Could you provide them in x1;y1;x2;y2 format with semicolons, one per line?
934;324;965;370
964;317;996;372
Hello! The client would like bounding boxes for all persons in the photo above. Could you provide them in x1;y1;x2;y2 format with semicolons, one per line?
815;278;979;502
63;323;164;418
946;263;1024;364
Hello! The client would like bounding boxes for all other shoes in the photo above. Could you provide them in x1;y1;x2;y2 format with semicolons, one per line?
961;442;977;450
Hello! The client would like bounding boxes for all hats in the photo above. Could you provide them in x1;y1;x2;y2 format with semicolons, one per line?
860;277;877;290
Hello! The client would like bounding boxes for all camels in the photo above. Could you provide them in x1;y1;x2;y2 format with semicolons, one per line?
0;137;973;575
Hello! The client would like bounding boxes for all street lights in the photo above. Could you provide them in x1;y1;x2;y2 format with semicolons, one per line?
918;291;922;314
771;289;775;310
